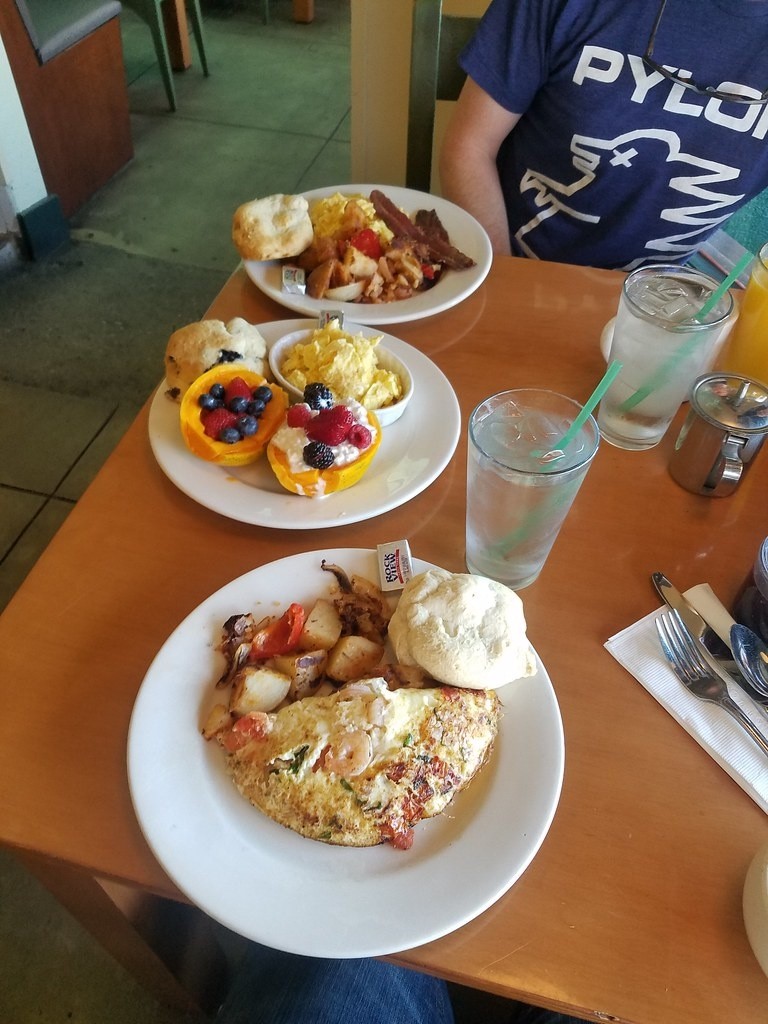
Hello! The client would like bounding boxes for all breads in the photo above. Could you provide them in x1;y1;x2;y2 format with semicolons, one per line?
164;315;277;398
232;193;313;262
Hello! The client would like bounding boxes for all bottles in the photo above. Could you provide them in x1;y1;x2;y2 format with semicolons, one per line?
730;535;767;644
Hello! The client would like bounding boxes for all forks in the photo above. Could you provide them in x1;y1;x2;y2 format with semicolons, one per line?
652;570;768;722
654;608;768;760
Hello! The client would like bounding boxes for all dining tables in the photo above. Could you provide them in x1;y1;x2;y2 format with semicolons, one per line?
3;251;768;1023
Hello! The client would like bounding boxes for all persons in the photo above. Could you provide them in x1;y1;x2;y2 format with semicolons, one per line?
438;0;767;268
163;913;452;1024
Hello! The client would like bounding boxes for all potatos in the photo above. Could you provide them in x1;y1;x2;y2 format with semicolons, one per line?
200;578;396;737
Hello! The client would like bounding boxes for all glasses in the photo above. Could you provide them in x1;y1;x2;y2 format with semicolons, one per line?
642;0;768;105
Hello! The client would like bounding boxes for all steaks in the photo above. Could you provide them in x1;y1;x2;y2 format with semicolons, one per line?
371;189;476;269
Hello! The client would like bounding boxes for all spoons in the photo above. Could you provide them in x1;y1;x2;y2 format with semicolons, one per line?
730;623;767;699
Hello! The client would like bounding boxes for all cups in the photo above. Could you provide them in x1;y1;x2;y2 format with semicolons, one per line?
463;388;601;591
668;370;768;497
726;240;767;400
595;264;734;452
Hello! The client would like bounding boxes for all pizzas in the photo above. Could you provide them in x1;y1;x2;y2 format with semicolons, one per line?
220;679;502;850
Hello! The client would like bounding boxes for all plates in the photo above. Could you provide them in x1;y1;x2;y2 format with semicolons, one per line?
269;330;414;427
147;319;462;529
126;547;567;958
240;184;493;324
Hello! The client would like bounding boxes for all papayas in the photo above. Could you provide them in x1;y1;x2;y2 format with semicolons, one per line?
182;364;381;495
